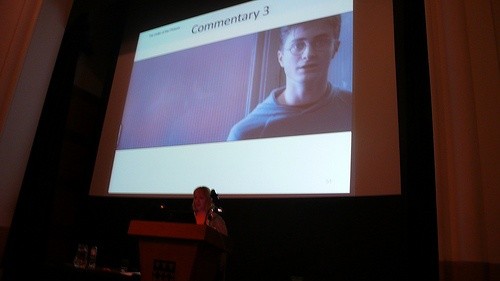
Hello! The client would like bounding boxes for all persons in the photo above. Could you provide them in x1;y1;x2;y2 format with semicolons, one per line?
225;14;353;142
185;186;228;281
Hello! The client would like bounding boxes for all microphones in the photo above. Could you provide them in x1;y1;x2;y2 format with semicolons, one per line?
208;214;212;227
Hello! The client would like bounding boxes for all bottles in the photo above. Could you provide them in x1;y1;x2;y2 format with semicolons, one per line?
74;242;98;269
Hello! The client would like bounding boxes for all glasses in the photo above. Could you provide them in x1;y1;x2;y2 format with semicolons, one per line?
282;37;340;56
